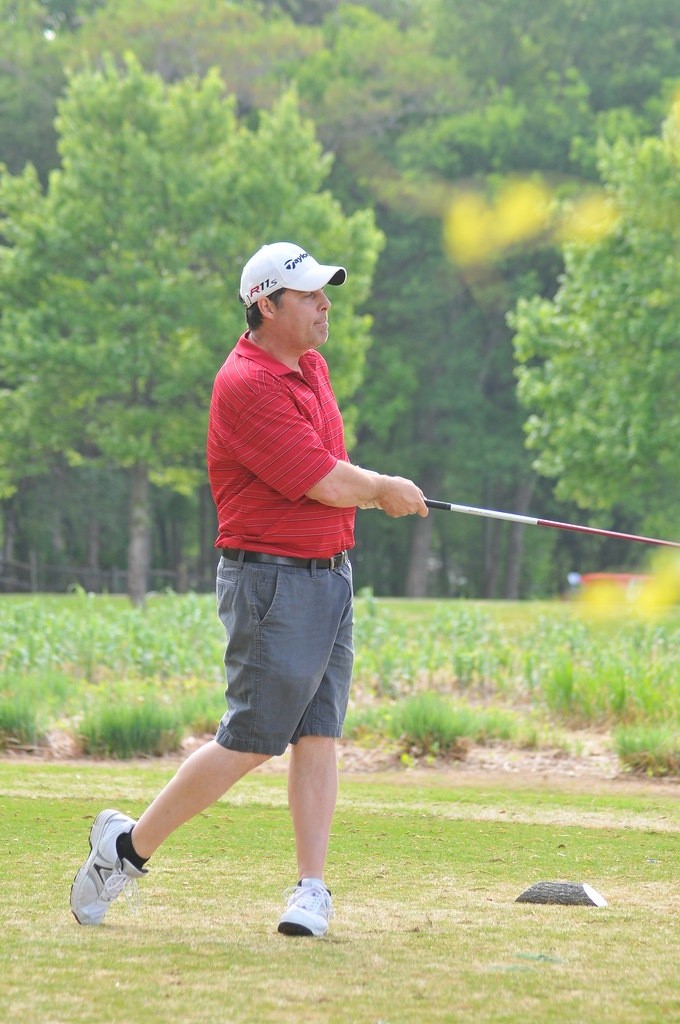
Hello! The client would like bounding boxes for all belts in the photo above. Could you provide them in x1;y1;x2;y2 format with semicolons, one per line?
222;547;348;569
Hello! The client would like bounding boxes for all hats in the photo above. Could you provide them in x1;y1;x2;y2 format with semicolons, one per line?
240;242;347;308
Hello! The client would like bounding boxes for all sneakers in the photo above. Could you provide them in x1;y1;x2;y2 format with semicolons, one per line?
69;809;149;926
277;878;334;937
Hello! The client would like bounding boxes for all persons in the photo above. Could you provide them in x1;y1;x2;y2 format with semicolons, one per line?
71;242;428;937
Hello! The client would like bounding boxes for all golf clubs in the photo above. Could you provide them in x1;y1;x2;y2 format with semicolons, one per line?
423;496;680;547
